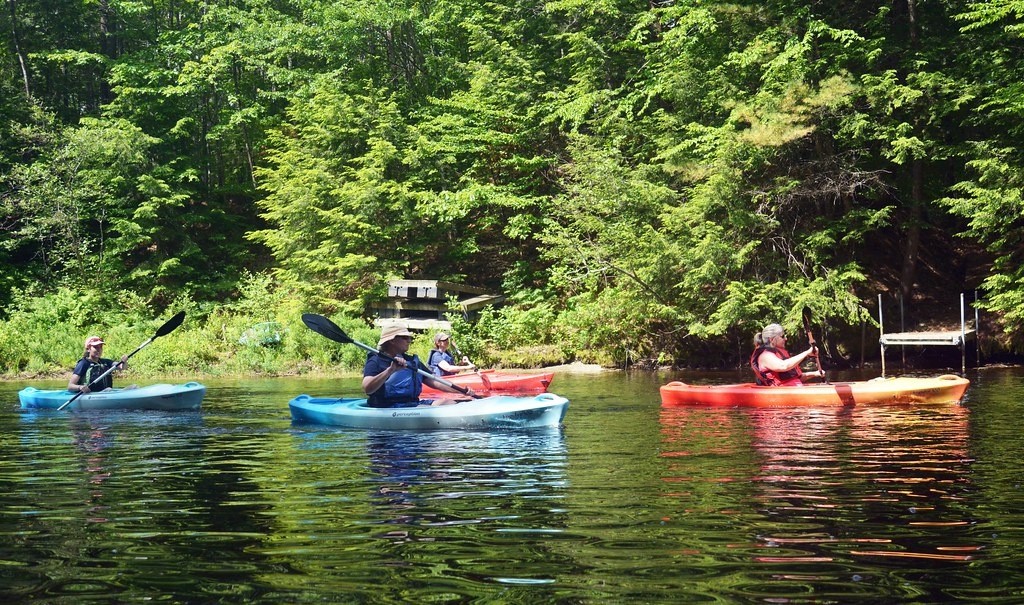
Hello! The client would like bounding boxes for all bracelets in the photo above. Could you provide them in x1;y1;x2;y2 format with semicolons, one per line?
78;386;80;391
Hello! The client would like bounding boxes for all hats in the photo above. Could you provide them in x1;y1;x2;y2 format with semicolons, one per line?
378;325;413;346
85;336;106;348
435;333;449;342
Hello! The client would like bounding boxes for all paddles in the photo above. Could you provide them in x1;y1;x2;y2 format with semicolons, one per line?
802;304;827;378
302;313;486;401
58;311;187;411
451;341;493;391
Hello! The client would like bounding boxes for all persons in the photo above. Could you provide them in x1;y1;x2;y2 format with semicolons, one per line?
428;332;475;376
362;324;475;408
751;323;825;387
68;337;128;392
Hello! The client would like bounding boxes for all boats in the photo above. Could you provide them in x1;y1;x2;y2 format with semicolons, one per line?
17;380;209;411
659;373;971;409
418;368;556;400
289;394;569;432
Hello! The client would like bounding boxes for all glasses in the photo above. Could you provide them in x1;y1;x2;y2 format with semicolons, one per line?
441;338;448;341
780;335;784;338
